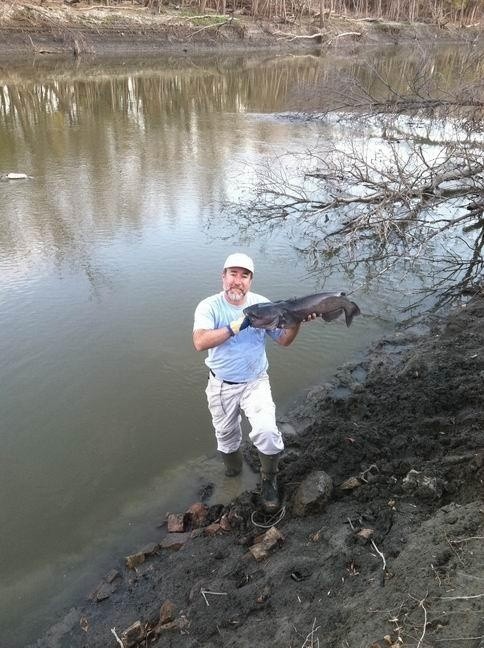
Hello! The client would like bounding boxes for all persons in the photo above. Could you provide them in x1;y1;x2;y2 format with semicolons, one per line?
192;252;323;513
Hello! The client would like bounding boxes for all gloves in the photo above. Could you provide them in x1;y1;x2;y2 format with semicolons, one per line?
226;313;252;337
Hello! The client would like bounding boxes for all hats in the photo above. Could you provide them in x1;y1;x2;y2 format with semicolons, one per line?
223;252;255;280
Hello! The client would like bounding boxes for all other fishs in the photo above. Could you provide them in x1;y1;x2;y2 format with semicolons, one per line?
242;290;361;331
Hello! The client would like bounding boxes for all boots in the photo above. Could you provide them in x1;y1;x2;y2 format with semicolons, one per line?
257;450;282;515
219;450;244;479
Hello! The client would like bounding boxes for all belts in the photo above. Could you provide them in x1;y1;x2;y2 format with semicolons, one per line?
209;369;247;385
